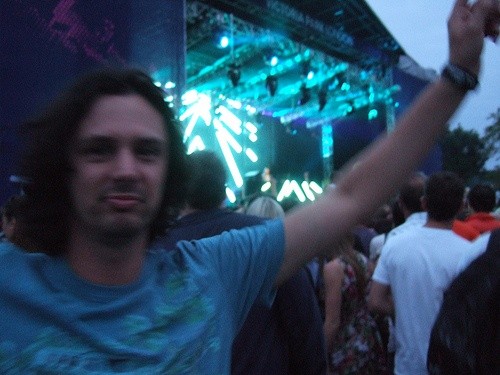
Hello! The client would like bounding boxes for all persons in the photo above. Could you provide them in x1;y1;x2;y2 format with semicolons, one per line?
160;150;500;375
0;0;497;375
0;195;21;240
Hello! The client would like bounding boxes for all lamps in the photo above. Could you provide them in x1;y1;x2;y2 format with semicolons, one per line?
265;76;277;97
317;92;327;113
226;63;241;86
299;87;310;107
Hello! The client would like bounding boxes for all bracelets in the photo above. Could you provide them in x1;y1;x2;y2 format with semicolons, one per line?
444;62;478;90
